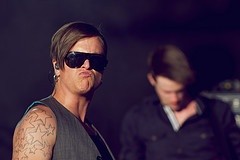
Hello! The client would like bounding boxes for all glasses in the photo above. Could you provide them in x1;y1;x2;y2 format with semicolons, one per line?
64;51;108;70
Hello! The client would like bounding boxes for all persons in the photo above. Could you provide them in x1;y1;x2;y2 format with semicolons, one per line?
119;45;240;160
11;22;116;160
